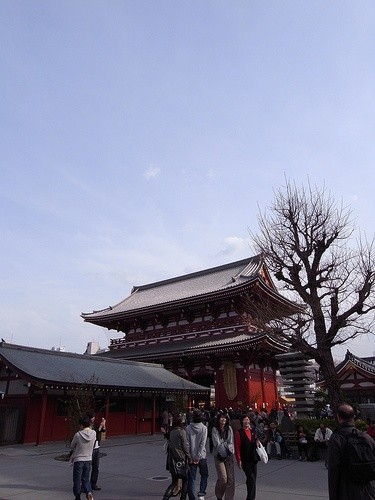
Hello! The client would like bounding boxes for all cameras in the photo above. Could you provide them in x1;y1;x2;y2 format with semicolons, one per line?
101;418;104;422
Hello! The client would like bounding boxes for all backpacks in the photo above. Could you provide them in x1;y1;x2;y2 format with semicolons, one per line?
338;428;375;482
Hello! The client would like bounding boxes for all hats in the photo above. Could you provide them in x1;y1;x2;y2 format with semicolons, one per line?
193;410;203;422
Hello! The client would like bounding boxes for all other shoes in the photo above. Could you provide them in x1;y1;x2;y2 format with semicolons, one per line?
92;488;101;490
88;491;94;500
198;496;205;500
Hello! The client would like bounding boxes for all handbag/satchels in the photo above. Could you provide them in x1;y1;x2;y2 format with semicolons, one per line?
168;446;188;480
256;440;268;464
217;448;232;462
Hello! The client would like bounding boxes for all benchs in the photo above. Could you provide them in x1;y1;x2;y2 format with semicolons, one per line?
281;431;308;458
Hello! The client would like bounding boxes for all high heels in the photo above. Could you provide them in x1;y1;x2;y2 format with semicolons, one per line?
170;487;181;497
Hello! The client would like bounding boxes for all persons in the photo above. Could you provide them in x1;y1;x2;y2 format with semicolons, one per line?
211;413;235;500
266;420;282;460
186;410;209;500
234;414;261;500
327;404;375;500
308;422;333;462
297;426;313;461
81;412;107;493
70;417;97;500
161;414;193;500
160;402;297;459
